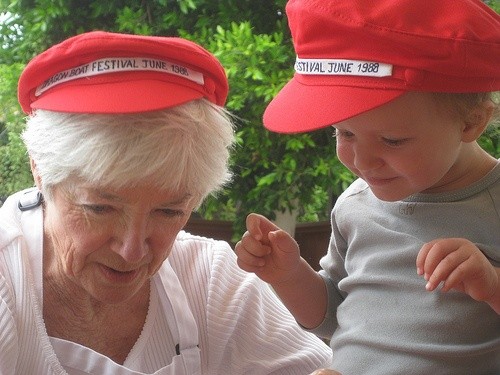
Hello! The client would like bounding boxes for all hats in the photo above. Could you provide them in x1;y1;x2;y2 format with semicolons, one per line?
17;29;230;117
260;0;500;135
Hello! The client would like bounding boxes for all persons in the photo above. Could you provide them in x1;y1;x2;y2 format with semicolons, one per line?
1;27;341;375
233;0;500;375
